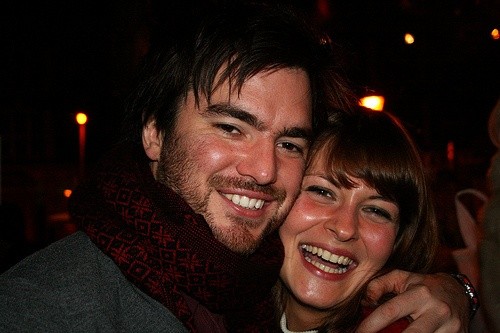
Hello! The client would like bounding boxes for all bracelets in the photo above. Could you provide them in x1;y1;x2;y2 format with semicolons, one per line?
446;273;479;320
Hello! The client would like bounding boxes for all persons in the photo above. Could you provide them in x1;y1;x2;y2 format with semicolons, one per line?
257;105;440;332
0;0;479;333
